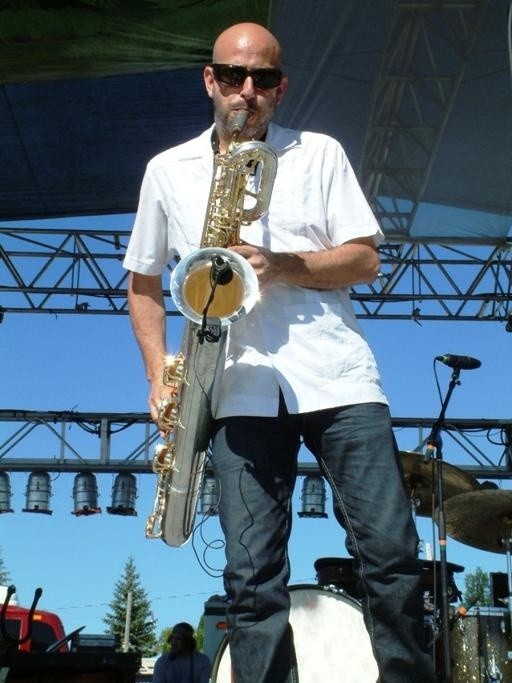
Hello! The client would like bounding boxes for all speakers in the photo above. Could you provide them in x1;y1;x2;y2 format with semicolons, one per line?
5;652;138;683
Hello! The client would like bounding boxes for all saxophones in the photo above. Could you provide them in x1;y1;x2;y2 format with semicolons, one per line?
146;109;277;546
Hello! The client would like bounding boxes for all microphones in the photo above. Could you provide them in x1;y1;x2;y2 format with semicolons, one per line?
436;354;482;369
212;255;233;284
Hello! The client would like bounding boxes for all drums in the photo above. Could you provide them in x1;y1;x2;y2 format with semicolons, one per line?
211;584;380;683
448;604;512;682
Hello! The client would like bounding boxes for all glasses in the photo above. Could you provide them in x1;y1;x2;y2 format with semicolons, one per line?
210;63;282;89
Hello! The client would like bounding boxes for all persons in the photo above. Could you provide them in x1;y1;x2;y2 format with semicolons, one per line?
149;620;213;683
119;19;444;683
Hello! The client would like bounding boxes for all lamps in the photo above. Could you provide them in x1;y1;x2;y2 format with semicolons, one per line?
0;469;137;516
200;471;219;515
298;473;328;519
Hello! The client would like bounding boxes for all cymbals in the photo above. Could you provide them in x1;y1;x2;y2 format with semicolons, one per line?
400;451;482;517
434;489;511;555
